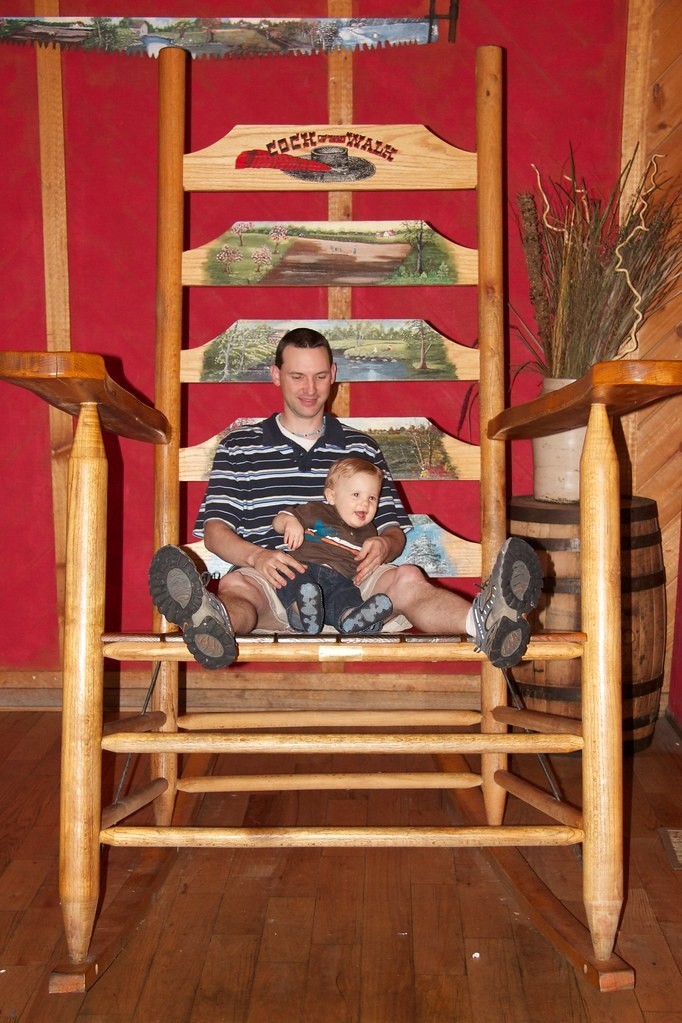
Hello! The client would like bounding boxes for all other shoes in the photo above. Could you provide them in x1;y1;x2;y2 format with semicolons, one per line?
296;580;324;635
340;593;393;634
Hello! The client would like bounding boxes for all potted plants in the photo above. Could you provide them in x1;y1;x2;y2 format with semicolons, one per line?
500;134;682;509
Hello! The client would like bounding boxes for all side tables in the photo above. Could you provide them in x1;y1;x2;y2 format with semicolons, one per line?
500;492;669;754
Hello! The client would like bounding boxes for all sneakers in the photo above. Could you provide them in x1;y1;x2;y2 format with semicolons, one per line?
472;536;544;669
147;544;239;669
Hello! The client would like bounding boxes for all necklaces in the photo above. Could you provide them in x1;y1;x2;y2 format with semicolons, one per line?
277;407;329;438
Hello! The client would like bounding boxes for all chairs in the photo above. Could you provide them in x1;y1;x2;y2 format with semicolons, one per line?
1;44;682;997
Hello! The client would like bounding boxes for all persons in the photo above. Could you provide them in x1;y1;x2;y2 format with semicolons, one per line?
270;458;394;638
147;327;544;674
